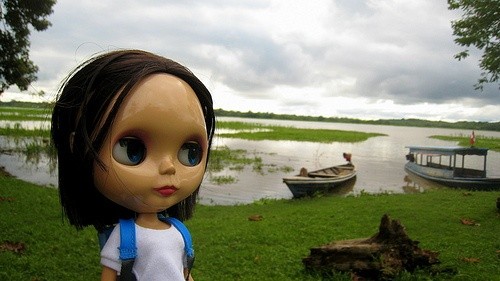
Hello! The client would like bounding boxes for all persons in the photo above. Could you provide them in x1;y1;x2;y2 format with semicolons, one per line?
48;49;215;281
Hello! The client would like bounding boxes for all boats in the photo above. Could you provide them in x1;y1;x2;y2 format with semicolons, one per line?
404;129;500;191
282;154;358;199
